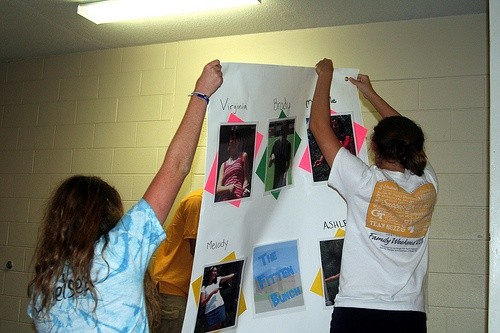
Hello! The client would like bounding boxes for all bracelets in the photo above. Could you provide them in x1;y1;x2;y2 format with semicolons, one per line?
186;90;210;104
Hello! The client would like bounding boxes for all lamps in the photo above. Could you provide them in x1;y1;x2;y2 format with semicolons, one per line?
75;0;262;26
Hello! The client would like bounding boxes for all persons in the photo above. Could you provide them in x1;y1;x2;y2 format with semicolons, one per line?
308;56;439;333
25;60;225;333
199;267;239;330
148;184;204;333
311;114;353;168
269;121;291;191
218;125;251;199
